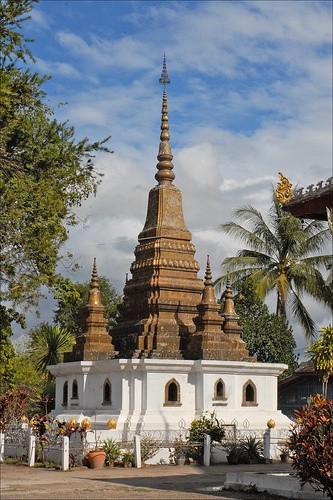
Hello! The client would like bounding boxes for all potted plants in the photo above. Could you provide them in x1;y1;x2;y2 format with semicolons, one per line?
242;436;264;464
87;451;106;470
174;445;185;465
225;446;240;464
104;437;121;468
123;453;133;468
279;448;288;462
